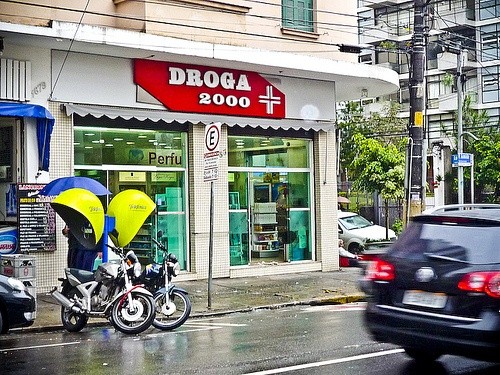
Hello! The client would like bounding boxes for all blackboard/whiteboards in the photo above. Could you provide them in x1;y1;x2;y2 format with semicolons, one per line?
18;185;57;252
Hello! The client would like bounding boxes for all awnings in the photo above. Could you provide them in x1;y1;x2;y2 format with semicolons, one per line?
0;102;55;172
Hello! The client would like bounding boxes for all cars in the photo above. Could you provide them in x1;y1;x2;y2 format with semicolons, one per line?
338;212;398;255
0;275;37;332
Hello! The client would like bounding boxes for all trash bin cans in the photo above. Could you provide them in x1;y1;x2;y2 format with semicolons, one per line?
2;256;37;323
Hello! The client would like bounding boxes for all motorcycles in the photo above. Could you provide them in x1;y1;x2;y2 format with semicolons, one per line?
49;228;191;335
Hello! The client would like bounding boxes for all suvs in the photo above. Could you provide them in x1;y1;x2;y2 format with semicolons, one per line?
357;204;500;361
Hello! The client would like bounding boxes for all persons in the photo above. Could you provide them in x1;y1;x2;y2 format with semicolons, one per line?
279;183;288;198
62;224;103;271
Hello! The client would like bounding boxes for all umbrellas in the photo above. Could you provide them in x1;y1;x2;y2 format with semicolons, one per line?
38;177;112;196
338;196;351;204
